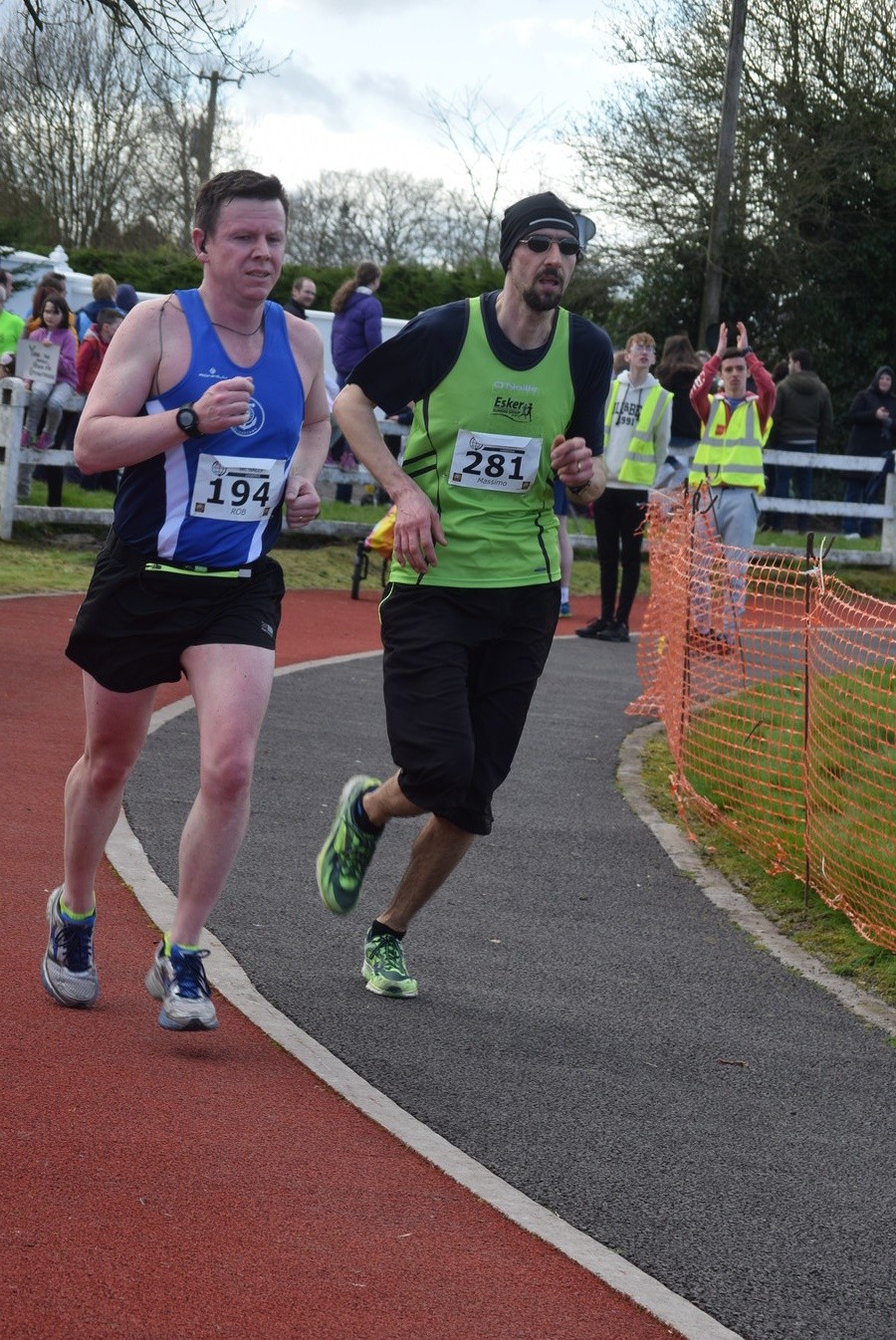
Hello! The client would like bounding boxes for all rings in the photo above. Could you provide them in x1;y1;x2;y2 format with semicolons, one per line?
298;516;303;522
578;462;582;472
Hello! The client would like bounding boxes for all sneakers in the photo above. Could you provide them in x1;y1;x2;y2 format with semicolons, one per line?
315;774;385;915
559;602;572;618
361;927;418;997
596;619;630;642
575;617;617;638
144;928;218;1032
41;880;99;1005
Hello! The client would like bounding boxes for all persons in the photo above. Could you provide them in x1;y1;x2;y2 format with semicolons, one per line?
316;190;612;998
842;364;896;542
0;259;414;507
45;170;332;1027
689;321;776;655
655;335;830;533
553;332;673;642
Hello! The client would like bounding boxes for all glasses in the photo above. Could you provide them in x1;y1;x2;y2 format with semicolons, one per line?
629;345;656;355
519;236;582;255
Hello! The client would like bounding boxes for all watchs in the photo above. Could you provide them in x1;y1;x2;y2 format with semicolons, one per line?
176;401;203;440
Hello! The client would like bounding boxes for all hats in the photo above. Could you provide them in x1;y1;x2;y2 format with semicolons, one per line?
115;283;138;310
499;192;579;273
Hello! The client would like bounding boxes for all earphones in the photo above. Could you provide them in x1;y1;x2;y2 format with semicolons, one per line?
201;241;206;254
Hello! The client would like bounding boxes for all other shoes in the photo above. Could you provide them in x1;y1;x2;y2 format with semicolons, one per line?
324;453;339;467
760;524;783;532
33;433;56;452
799;527;814;536
689;627;734;656
844;532;861;540
19;430;33;450
339;452;359;472
